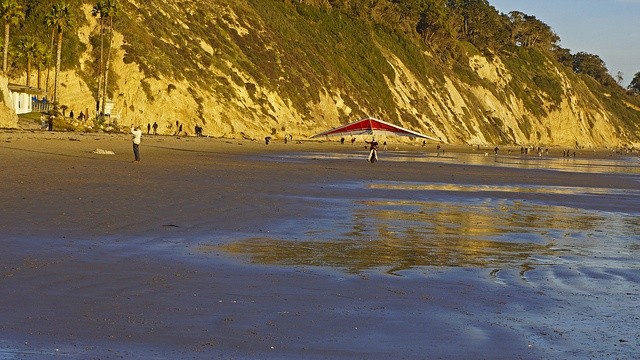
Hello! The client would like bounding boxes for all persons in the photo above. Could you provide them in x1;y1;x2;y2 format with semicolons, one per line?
339;137;362;143
39;109;86;133
264;133;294;144
365;134;379;162
421;138;445;157
491;144;577;158
146;120;204;136
130;123;143;164
